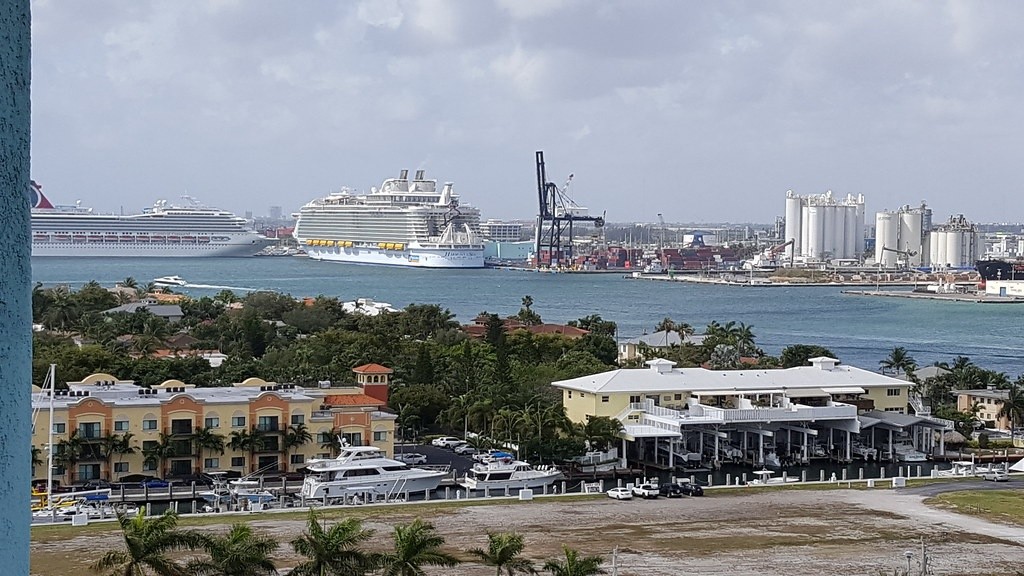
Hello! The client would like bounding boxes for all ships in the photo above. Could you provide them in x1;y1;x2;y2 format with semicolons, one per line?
291;169;486;269
31;182;271;257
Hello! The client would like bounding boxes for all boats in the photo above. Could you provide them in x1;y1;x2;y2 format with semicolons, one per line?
932;460;1005;476
201;469;277;514
295;434;451;501
747;469;800;484
459;461;562;491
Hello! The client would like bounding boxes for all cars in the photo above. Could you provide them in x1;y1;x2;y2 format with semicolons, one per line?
606;487;633;500
432;436;515;465
981;471;1010;482
395;453;427;465
680;484;704;496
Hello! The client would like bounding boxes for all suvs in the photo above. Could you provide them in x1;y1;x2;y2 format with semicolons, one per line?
658;483;684;498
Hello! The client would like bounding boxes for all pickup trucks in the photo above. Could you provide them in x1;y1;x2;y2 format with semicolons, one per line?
631;484;660;500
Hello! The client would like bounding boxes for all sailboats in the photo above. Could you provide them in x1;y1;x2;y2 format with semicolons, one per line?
32;363;139;524
